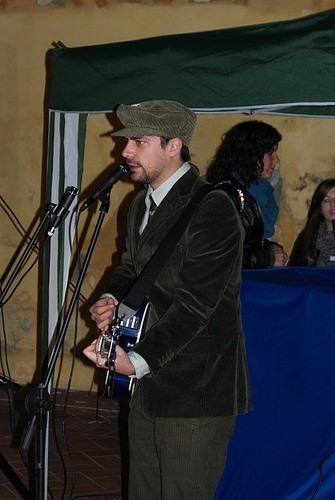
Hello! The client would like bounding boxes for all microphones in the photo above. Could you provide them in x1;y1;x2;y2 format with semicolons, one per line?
80;162;131;212
47;203;56;211
52;186;79;228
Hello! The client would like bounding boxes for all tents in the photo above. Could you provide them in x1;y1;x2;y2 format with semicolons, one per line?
37;8;335;500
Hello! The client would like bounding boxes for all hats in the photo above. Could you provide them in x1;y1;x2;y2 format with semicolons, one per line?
110;99;198;149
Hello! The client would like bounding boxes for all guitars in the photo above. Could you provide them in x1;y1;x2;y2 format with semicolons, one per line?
96;302;150;402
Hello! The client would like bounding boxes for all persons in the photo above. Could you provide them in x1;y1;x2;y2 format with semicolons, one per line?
286;178;335;268
83;100;252;500
205;120;288;268
